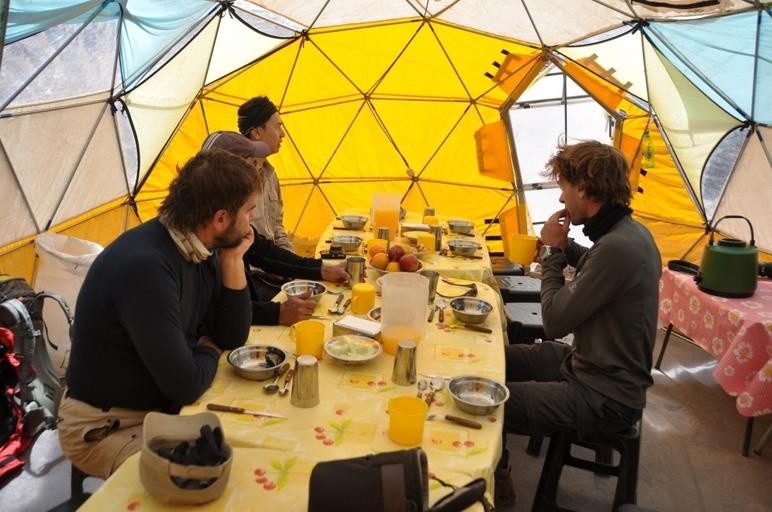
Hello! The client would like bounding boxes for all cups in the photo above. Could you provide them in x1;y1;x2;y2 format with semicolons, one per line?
381;272;429;385
421;207;440;226
368;195;400;251
347;257;365;290
352;283;376;315
290;319;325;408
416;232;436;258
388;395;427;447
509;234;538;267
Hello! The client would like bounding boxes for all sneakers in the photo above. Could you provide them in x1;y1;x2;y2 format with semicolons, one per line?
495;467;517;509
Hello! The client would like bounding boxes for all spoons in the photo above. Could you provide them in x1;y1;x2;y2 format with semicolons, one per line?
264;363;293;396
417;376;445;408
428;298;447;323
328;294;351;314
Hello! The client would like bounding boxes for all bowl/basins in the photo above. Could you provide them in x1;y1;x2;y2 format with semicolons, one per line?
448;374;510;415
450;297;493;324
282;280;325;303
229;344;287;381
331;235;362;252
342;216;368;231
447;220;474;234
447;240;478;255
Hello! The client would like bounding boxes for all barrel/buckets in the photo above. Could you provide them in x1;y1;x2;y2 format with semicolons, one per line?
378;271;430;354
694;215;758;298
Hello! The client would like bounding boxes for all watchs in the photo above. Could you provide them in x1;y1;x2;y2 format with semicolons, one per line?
539;245;562;260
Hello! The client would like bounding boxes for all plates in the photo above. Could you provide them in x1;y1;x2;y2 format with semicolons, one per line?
368;258;425;276
325;334;381;363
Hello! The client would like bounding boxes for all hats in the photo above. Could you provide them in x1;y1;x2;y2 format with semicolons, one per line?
236;96;278;134
202;130;271;159
141;411;234;506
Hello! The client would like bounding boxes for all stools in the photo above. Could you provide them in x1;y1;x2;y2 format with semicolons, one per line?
70;467;105;509
491;257;641;512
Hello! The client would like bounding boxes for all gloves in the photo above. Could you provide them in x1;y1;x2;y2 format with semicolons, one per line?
170;424;226;467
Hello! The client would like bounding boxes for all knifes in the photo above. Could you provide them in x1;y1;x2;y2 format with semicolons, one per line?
207;403;288;419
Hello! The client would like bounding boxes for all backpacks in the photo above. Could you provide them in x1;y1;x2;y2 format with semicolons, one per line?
0;273;74;479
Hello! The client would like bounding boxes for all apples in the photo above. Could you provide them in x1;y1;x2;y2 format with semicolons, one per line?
389;245;405;260
399;254;419;270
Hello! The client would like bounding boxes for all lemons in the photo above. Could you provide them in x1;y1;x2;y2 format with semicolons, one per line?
369;246;400;271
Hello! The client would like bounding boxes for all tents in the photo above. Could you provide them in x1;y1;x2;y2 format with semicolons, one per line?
0;0;772;290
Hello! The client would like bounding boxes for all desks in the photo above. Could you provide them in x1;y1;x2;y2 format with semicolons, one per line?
654;268;771;457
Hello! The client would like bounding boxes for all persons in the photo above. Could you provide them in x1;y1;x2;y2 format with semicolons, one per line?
58;146;262;480
202;131;368;326
239;96;294;302
494;139;662;511
